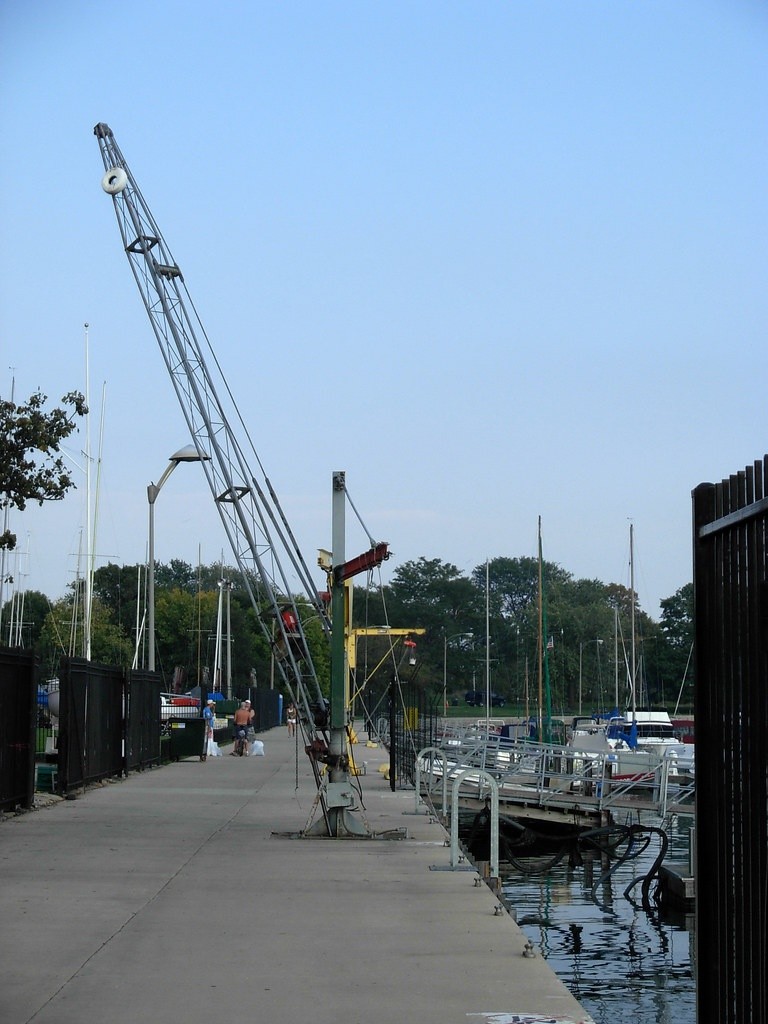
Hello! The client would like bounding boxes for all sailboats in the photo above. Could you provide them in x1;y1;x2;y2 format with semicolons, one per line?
407;514;697;840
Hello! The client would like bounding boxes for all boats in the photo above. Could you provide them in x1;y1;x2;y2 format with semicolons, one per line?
45;675;202;725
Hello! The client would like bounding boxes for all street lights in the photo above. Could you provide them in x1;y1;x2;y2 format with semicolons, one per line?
144;444;215;671
217;577;239;699
349;626;391;722
443;633;477;720
270;602;317;692
579;639;605;716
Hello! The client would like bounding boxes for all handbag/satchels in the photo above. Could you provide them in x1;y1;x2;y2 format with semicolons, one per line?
210;741;222;757
251;740;265;756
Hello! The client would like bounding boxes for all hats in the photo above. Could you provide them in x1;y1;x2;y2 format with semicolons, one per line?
245;700;251;704
207;699;216;704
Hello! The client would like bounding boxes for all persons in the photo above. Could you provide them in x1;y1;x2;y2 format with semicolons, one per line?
229;700;255;757
286;703;296;738
203;699;215;755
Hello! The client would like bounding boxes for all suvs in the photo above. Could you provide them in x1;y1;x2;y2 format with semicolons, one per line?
466;690;506;708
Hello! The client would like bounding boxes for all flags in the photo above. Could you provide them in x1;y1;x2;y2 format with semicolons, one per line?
546;635;554;648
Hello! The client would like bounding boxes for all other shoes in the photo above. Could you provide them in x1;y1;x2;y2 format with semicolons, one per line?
246;750;249;756
288;735;291;738
202;753;207;757
229;750;237;755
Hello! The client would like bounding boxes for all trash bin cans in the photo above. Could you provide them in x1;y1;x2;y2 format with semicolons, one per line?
167;717;209;762
